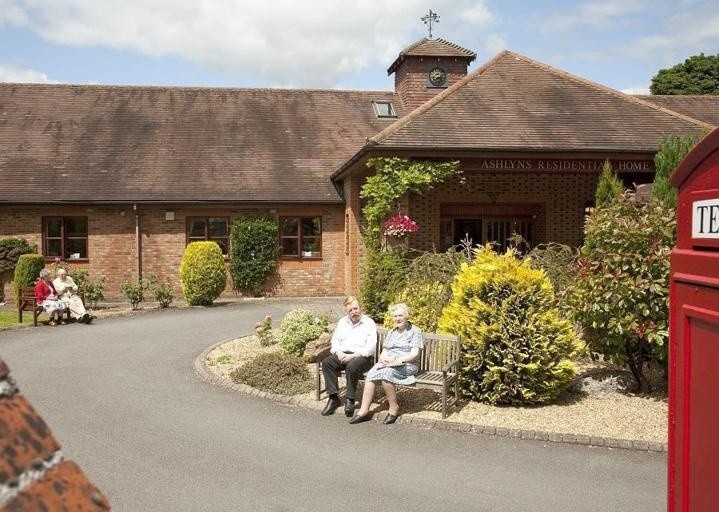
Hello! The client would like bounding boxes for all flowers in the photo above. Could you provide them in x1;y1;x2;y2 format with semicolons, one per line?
384;215;419;238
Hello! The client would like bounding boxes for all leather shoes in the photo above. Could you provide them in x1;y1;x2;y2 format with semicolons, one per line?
322;398;341;416
383;413;397;424
345;398;372;424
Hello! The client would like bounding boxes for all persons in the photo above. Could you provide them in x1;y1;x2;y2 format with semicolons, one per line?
34;267;68;327
320;295;378;417
348;302;425;425
52;269;95;324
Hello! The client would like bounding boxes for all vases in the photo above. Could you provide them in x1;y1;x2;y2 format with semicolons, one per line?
396;233;407;239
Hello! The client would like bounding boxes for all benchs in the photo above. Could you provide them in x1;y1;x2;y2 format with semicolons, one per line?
17;287;84;327
313;327;461;419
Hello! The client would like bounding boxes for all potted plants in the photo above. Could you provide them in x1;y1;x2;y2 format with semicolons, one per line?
302;239;311;256
312;239;321;257
